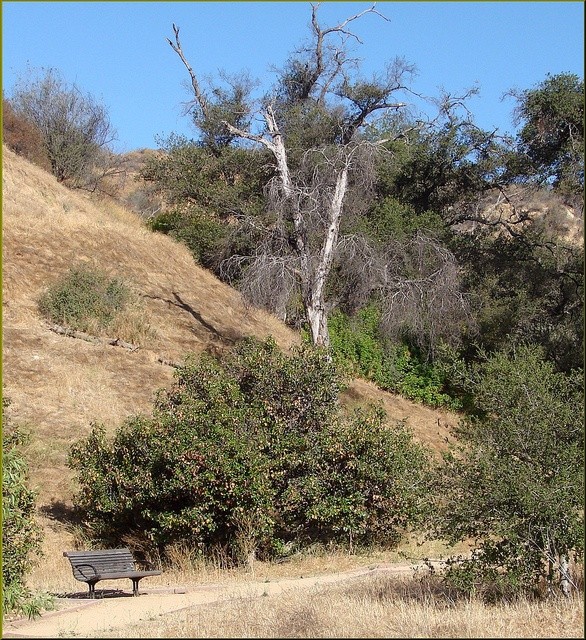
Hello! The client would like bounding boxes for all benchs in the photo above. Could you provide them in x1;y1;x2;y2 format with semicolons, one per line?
64;548;161;600
